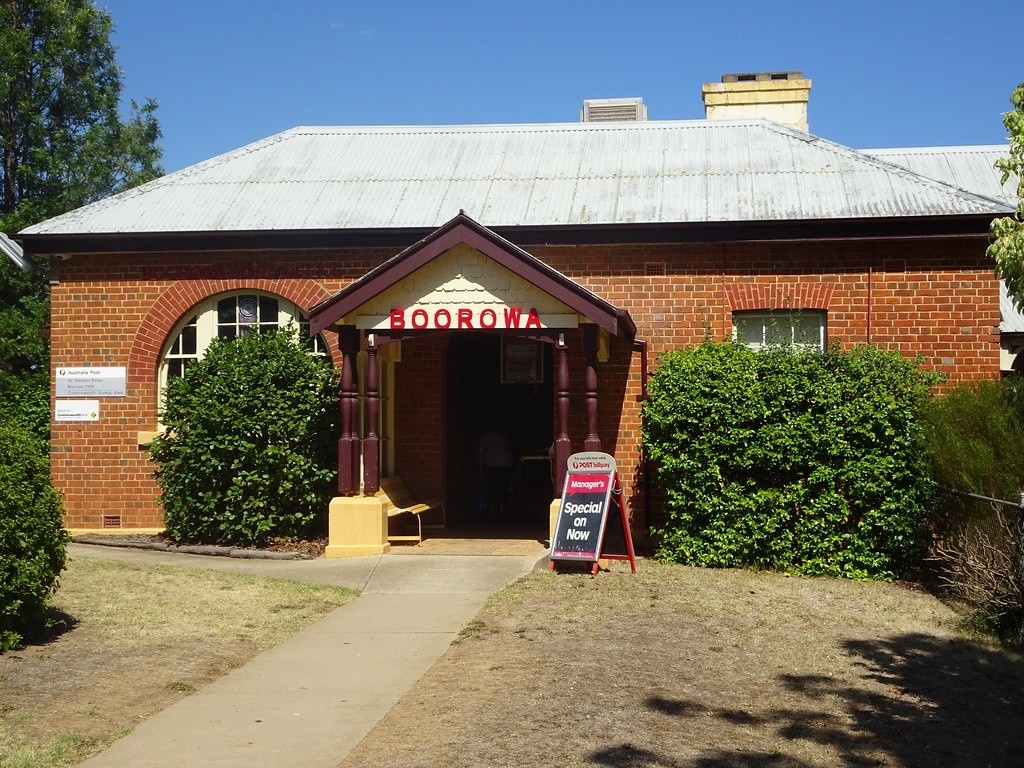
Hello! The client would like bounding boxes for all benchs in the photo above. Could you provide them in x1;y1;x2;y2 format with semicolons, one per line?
373;475;447;547
